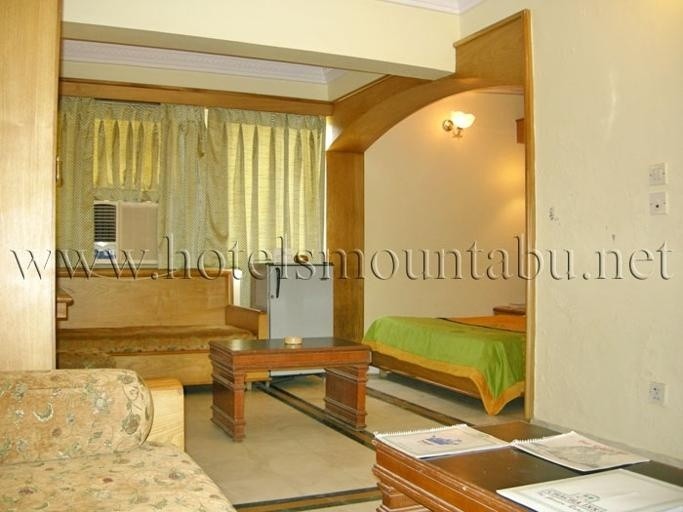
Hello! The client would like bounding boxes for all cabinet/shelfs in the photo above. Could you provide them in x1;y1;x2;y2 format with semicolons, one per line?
249;262;332;377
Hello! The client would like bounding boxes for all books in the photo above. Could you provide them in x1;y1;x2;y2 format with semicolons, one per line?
495;467;682;511
512;428;650;473
374;422;510;459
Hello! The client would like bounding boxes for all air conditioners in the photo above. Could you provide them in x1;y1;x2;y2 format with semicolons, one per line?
92;200;157;262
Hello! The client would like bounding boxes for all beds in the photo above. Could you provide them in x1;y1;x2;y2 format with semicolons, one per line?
361;304;525;415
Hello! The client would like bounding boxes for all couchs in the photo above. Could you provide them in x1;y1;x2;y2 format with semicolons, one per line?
55;267;268;385
0;370;234;510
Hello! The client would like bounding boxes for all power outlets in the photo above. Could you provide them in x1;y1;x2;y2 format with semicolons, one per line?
648;381;666;404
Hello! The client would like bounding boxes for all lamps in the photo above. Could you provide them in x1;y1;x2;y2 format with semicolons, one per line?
441;111;474;139
